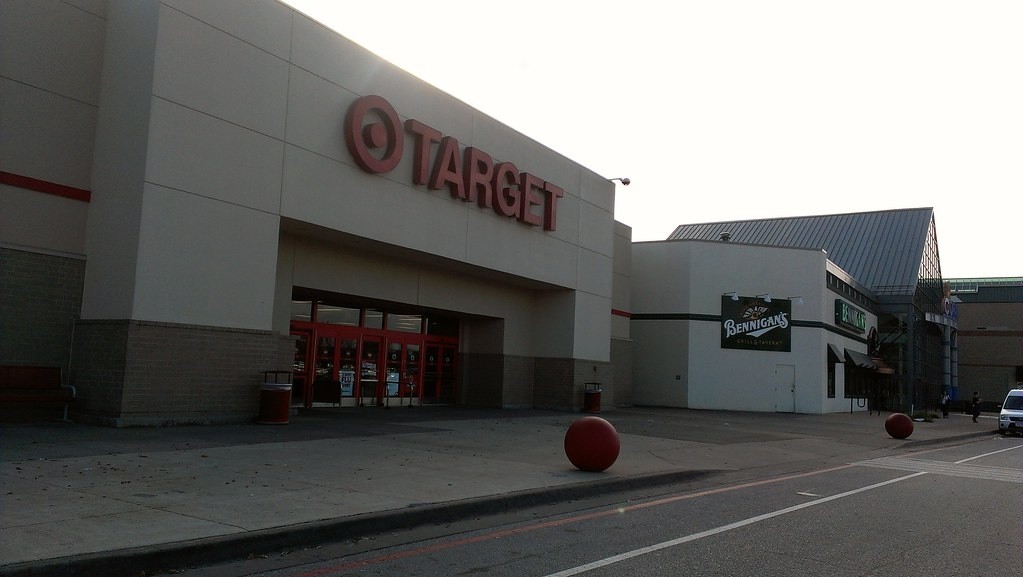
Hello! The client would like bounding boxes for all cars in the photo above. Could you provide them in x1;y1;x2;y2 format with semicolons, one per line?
996;389;1023;435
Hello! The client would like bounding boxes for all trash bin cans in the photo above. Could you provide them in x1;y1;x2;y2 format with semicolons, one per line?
259;370;294;425
584;382;603;414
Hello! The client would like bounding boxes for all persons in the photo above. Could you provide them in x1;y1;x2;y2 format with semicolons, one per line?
971;392;981;423
940;389;951;419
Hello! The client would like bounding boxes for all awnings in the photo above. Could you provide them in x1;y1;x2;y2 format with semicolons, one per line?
843;347;877;371
